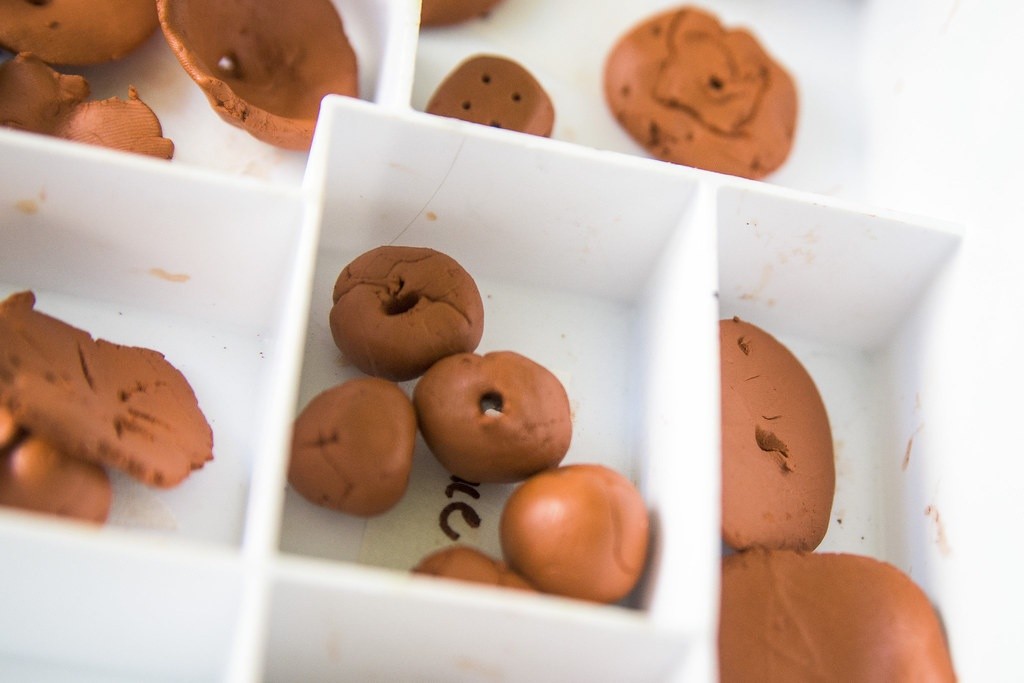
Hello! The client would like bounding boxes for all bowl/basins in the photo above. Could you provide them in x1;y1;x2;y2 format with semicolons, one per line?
0;0;1020;682
